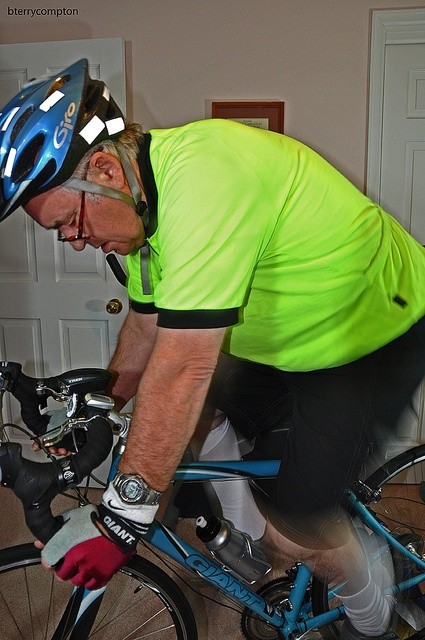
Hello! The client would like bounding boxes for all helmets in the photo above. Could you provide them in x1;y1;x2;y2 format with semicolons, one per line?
0;58;126;223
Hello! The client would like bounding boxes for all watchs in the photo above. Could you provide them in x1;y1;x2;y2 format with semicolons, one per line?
112;471;163;506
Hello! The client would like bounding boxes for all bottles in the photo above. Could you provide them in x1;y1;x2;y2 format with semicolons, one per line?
193;514;272;587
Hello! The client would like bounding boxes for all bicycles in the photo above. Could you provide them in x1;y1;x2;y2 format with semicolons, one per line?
0;360;424;639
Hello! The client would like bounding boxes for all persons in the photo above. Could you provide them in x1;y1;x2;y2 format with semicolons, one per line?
0;57;425;640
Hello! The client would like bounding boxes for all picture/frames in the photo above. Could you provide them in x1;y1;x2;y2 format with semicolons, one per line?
210;100;285;134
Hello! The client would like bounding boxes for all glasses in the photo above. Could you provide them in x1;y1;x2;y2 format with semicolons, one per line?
57;146;104;242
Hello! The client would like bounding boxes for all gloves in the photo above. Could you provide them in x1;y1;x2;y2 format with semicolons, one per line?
40;481;160;591
37;407;88;453
